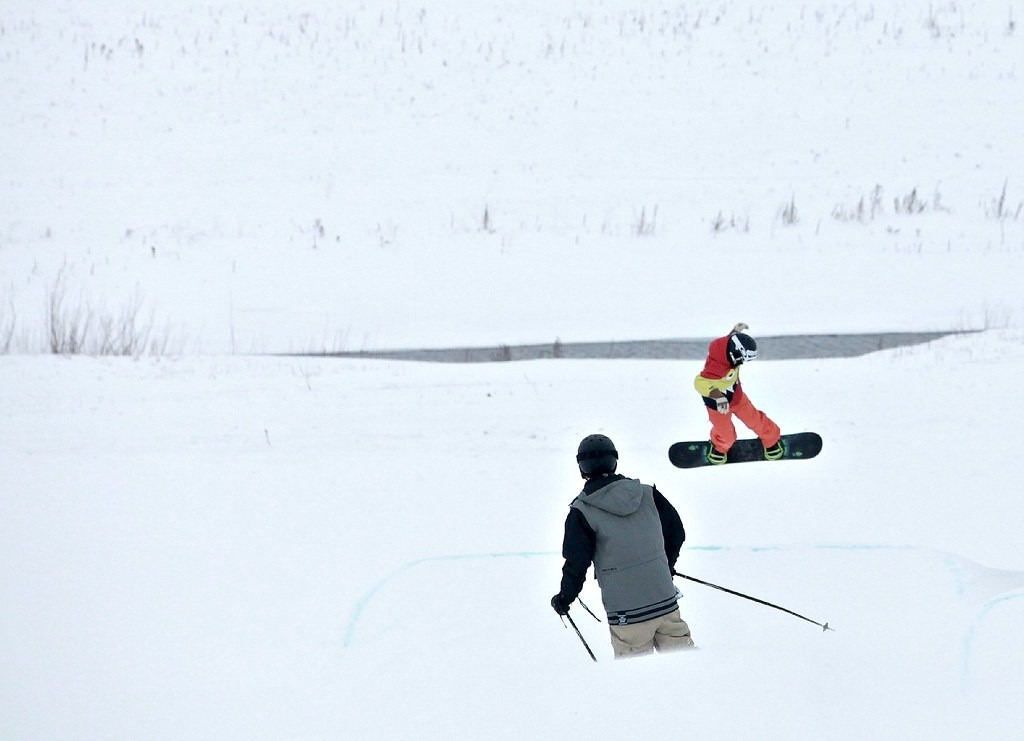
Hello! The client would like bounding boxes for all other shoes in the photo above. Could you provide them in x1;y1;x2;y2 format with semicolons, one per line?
765;442;780;456
711;449;724;460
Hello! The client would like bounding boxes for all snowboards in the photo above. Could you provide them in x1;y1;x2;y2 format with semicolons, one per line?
669;432;823;469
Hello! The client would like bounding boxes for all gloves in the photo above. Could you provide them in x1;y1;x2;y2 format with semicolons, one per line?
710;389;730;414
730;323;748;333
552;594;574;615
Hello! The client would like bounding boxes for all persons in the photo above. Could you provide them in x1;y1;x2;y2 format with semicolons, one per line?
694;322;784;464
550;434;698;660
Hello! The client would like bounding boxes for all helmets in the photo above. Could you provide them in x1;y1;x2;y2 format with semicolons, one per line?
728;334;757;366
578;434;618;475
